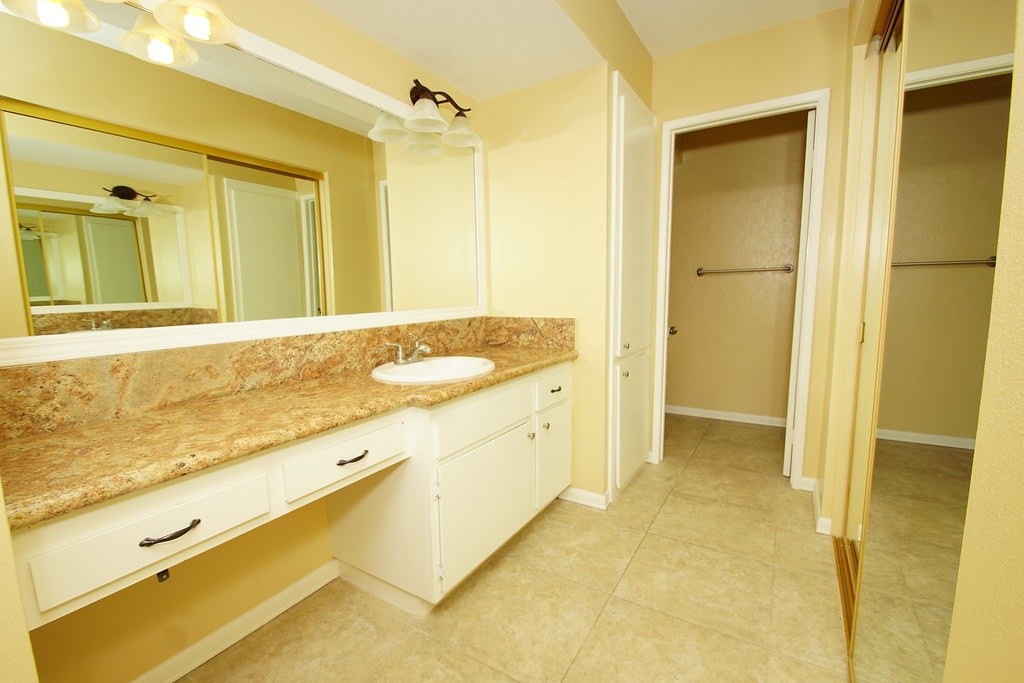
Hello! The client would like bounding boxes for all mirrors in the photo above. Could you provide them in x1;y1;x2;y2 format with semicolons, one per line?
3;110;336;337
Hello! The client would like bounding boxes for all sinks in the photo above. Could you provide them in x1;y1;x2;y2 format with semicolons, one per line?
371;355;497;385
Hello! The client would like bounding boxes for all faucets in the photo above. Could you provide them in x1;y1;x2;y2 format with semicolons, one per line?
405;337;433;364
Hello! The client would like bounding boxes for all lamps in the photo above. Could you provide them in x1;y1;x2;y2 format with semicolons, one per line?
403;79;478;150
153;0;239;45
118;10;201;70
366;110;441;153
1;0;102;34
